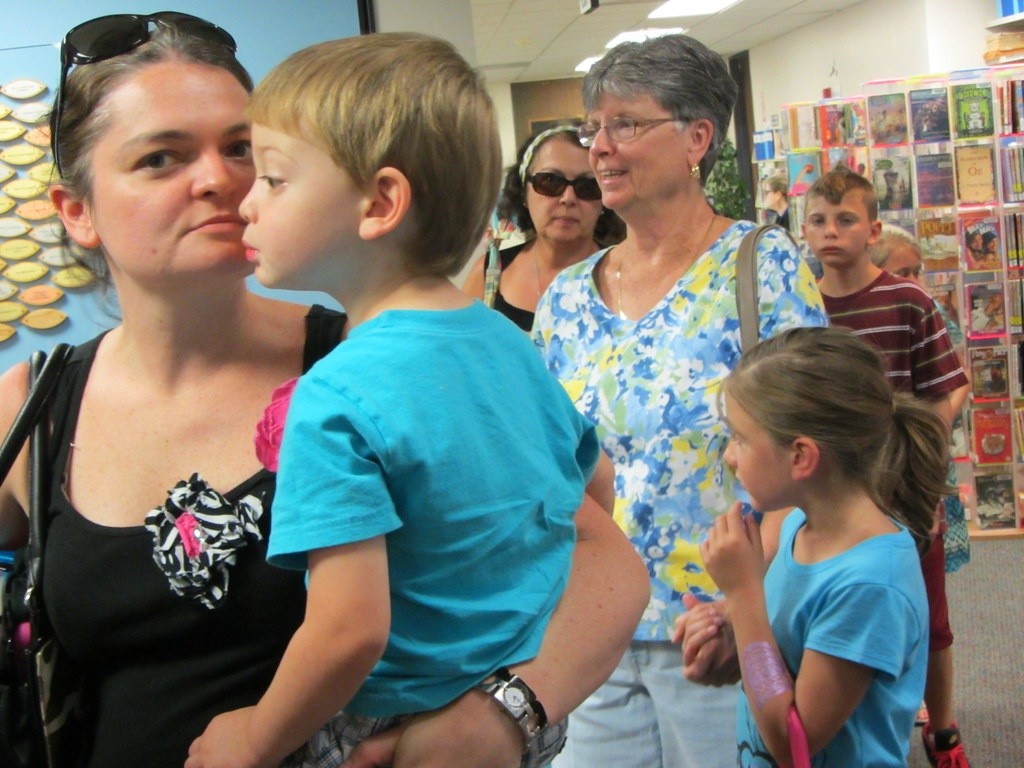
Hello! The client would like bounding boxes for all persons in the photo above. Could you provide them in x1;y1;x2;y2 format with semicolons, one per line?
0;14;652;766
671;325;949;766
526;35;830;768
800;164;973;768
181;30;622;764
459;115;626;329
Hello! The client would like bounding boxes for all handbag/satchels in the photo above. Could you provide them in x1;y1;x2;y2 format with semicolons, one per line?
0;341;87;768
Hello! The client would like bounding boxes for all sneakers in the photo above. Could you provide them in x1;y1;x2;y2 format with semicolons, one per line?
920;719;973;768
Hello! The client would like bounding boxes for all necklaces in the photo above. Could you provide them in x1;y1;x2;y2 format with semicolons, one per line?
616;214;716;318
532;242;553;298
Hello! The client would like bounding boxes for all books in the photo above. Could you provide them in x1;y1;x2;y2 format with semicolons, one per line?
753;68;1023;537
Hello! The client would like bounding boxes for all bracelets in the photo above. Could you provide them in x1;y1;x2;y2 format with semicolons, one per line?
475;667;547;752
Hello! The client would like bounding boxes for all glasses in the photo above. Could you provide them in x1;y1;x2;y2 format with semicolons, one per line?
52;12;237;179
576;116;687;146
525;172;603;201
